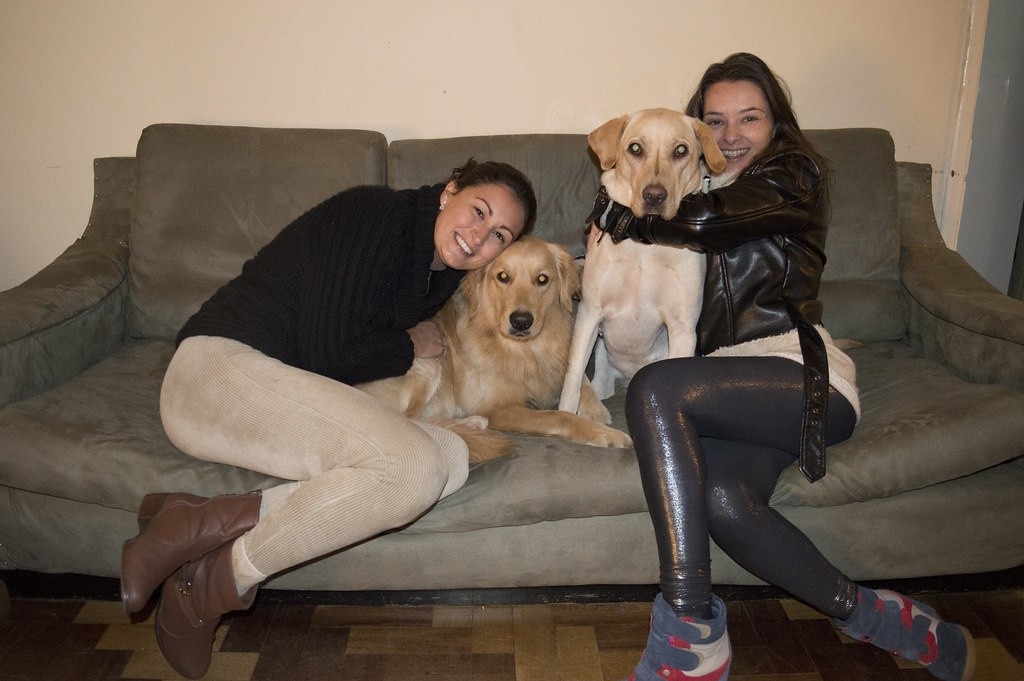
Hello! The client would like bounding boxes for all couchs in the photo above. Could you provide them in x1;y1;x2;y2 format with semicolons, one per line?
0;123;1024;592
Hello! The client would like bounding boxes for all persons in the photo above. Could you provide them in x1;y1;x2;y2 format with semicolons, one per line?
122;162;531;681
585;52;971;679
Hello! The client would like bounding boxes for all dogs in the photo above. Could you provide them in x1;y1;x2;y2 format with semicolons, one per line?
559;106;727;414
352;235;634;462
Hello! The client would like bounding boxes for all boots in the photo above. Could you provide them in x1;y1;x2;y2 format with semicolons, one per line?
833;584;976;681
624;593;733;681
152;532;261;680
120;489;264;615
